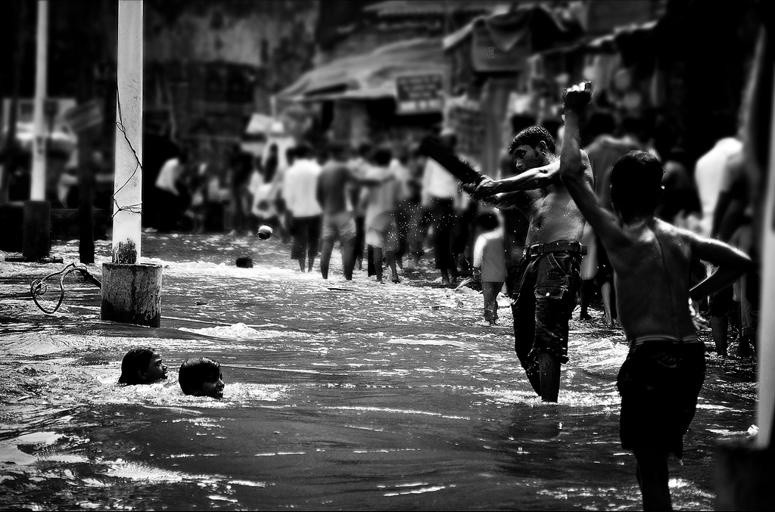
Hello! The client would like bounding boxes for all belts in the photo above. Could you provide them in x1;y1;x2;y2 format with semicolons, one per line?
527;240;588;258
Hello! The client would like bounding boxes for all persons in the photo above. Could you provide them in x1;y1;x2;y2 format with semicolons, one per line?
558;82;754;511
574;111;767;352
178;356;224;401
117;345;170;386
455;123;593;405
151;130;502;288
